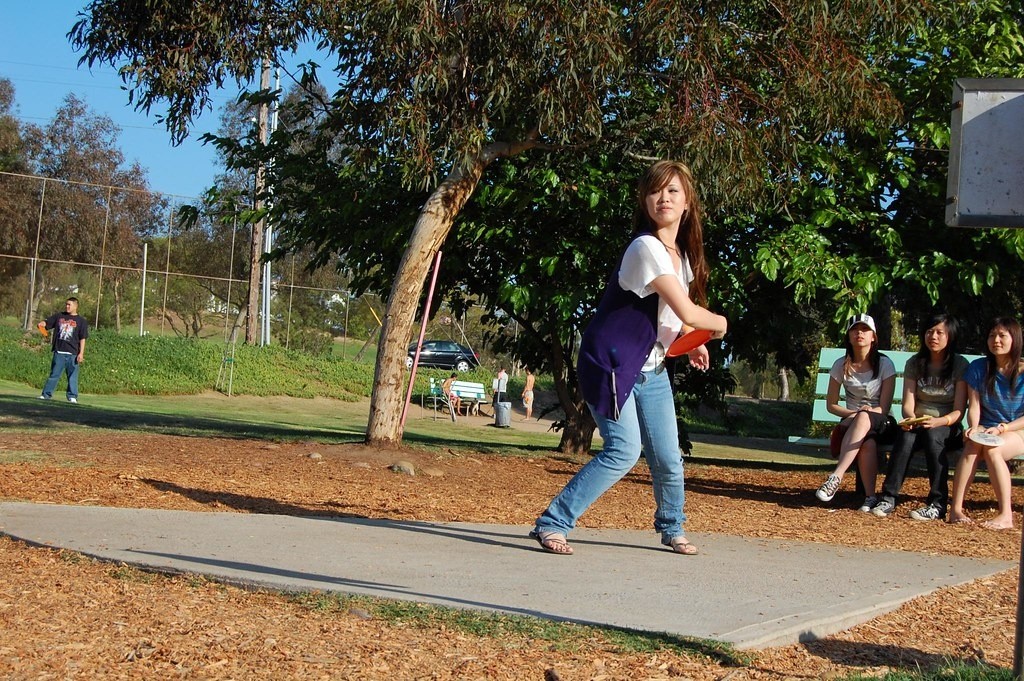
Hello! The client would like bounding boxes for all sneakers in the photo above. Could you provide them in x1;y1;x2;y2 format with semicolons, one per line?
874;496;895;516
816;474;842;501
909;503;942;520
857;495;877;512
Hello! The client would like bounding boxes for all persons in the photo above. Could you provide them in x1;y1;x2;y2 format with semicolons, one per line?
871;314;970;521
816;313;898;513
521;365;535;421
37;297;89;403
491;363;508;418
442;372;464;416
950;314;1024;530
528;161;727;555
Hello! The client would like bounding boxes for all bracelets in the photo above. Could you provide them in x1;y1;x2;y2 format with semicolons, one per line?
997;422;1008;434
680;325;693;335
944;414;953;426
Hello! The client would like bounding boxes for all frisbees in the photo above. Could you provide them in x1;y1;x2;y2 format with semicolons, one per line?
39;325;48;336
969;432;1005;447
900;415;933;426
666;329;716;359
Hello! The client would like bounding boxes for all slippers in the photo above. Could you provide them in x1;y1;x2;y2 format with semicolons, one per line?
661;535;699;555
530;526;573;555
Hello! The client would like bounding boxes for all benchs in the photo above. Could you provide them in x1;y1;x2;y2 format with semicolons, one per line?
426;378;494;419
788;348;1024;509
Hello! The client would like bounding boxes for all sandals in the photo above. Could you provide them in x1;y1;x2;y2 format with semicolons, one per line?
953;517;975;524
980;521;1012;531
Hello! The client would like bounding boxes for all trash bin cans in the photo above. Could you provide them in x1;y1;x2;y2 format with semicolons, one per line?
492;402;512;427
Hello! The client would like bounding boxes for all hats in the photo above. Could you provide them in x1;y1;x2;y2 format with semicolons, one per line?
846;313;875;334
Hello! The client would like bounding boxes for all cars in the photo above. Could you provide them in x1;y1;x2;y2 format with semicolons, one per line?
405;340;480;372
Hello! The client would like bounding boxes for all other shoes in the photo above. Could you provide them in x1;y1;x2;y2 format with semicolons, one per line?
70;398;77;402
38;395;44;399
457;413;462;416
520;417;530;421
